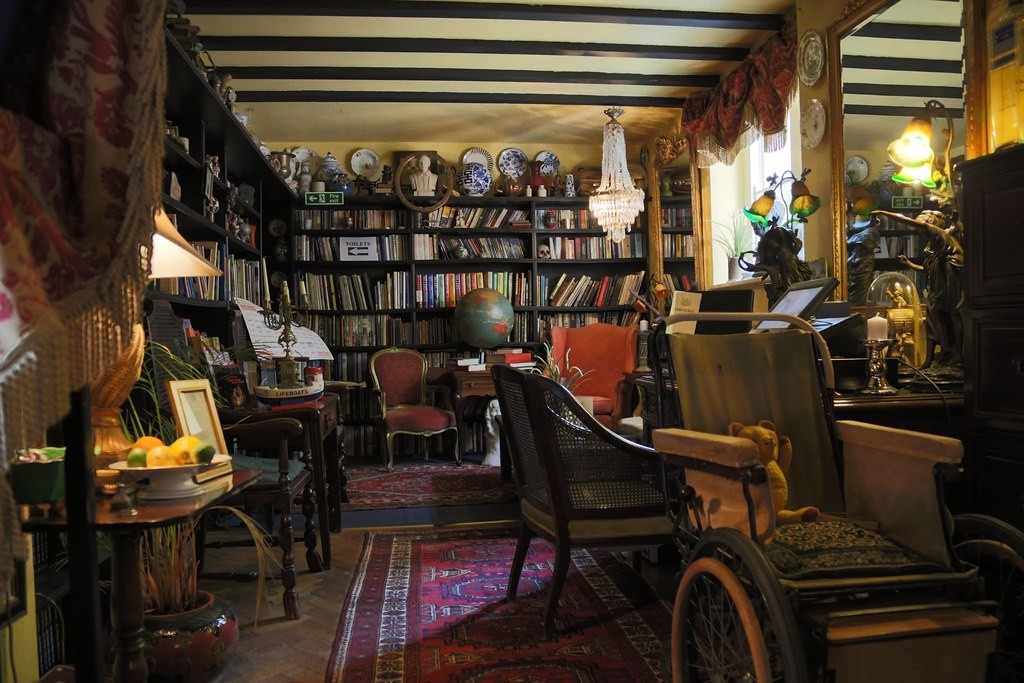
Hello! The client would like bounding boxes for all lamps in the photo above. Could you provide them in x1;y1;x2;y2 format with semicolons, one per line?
91;201;224;495
743;170;821;232
589;106;644;244
886;99;959;226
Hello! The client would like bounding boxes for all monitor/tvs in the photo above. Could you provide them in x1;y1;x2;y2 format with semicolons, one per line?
747;276;840;335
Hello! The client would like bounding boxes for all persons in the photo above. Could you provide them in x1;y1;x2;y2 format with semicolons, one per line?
870;210;964;370
768;227;803;289
409;155;438;190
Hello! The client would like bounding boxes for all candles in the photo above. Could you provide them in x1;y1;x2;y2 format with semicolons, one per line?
867;311;888;339
283;280;291;301
259;253;272;308
299;280;306;295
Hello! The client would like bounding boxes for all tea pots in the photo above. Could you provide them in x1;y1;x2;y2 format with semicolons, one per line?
495;181;524;198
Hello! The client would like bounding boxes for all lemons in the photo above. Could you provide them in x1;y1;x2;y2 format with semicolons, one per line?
127;436;216;469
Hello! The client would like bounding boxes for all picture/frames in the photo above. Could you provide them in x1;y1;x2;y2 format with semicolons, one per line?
392;151;438;191
164;377;235;482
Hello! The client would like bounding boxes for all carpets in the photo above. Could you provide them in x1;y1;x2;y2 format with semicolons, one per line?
287;455;509;512
323;529;705;683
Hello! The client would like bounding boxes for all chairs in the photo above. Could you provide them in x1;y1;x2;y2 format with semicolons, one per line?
145;297;1005;683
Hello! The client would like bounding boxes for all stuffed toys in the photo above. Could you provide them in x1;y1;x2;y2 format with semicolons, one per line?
727;419;819;525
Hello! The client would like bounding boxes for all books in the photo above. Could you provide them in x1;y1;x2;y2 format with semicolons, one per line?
160;213;262;307
297;206;695;457
867;213;927;303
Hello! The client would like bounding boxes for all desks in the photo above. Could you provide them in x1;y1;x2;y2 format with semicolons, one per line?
17;469;262;683
627;370;963;482
449;371;501;462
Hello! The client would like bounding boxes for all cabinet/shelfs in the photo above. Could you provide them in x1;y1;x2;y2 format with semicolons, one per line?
953;143;1024;592
659;196;695;295
158;28;647;466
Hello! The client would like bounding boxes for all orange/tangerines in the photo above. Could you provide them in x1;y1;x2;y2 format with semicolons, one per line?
134;437;165;452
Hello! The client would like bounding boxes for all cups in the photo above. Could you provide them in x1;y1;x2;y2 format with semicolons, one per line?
271;151;291;178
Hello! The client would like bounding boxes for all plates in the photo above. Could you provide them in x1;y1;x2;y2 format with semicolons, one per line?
535;151;560;177
800;98;826;151
844;156;868;182
350;148;380;178
289;147;322;178
461;148;494;173
795;29;825;88
498;148;528;178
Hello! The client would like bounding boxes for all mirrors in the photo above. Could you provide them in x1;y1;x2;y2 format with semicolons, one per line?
648;133;710;331
825;0;972;353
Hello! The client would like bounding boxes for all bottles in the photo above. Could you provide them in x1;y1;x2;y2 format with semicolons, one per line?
316;151;342;192
299;162;312;197
525;161;548;196
881;162;895;182
329;174;354;199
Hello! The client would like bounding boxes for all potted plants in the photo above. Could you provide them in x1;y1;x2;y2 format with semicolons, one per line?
47;311;291;683
532;341;594;437
